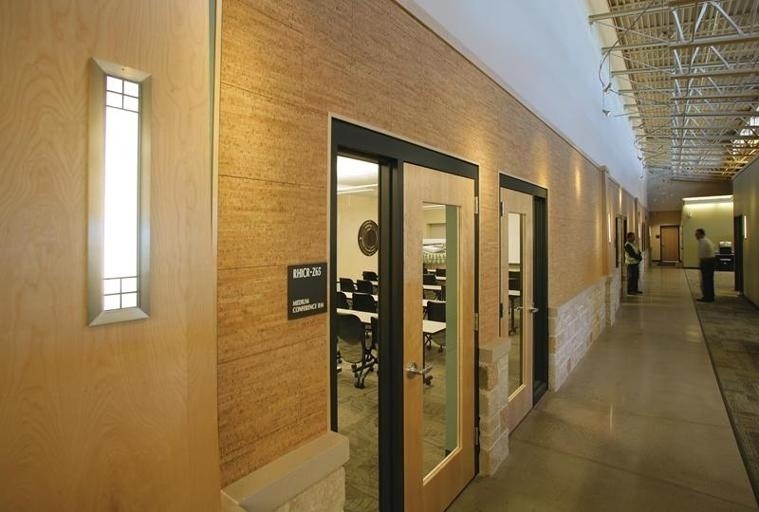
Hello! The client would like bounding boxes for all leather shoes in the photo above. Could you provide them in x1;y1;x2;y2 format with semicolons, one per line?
628;290;643;295
696;296;714;301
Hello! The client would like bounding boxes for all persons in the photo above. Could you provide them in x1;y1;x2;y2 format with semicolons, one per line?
694;228;716;302
623;231;644;296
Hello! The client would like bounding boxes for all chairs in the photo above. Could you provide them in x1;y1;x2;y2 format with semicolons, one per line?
337;269;520;390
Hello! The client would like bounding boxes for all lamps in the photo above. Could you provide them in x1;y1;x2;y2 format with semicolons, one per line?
599;50;611;117
